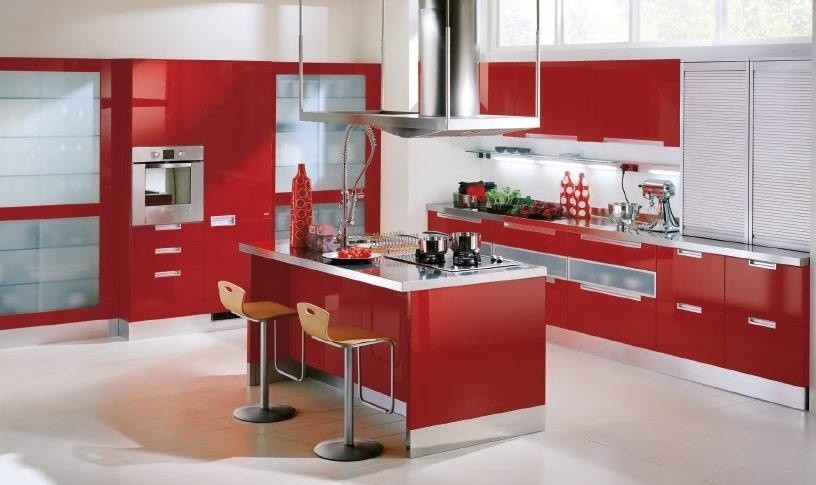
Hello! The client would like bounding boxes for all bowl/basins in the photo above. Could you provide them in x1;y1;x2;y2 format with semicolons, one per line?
608;202;639;225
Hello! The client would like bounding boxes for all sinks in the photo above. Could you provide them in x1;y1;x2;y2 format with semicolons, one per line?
331;236;404;244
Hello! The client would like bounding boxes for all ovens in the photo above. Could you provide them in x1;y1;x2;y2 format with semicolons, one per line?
129;145;206;227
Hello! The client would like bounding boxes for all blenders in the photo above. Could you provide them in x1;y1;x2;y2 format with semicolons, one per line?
637;180;679;232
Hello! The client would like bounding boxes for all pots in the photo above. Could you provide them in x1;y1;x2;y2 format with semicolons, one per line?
419;230;449;254
451;231;482;254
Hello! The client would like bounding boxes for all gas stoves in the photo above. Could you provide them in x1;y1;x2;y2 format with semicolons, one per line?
385;252;517;272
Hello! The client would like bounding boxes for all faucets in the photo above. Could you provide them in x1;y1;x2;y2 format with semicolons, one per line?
338;123;378;248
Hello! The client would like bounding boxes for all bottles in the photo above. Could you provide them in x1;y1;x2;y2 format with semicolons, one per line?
290;164;312;249
559;171;591;221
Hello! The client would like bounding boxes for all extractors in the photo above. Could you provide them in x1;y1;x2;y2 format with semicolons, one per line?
299;0;540;140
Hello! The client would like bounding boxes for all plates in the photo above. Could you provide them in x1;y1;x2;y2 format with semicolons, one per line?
322;252;381;259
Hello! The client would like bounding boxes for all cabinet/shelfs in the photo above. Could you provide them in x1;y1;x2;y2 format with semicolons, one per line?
656;244;816;388
474;60;680;146
679;58;811;254
427;210;656;353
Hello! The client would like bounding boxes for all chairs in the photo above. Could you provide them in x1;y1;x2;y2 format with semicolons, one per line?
217;279;399;462
